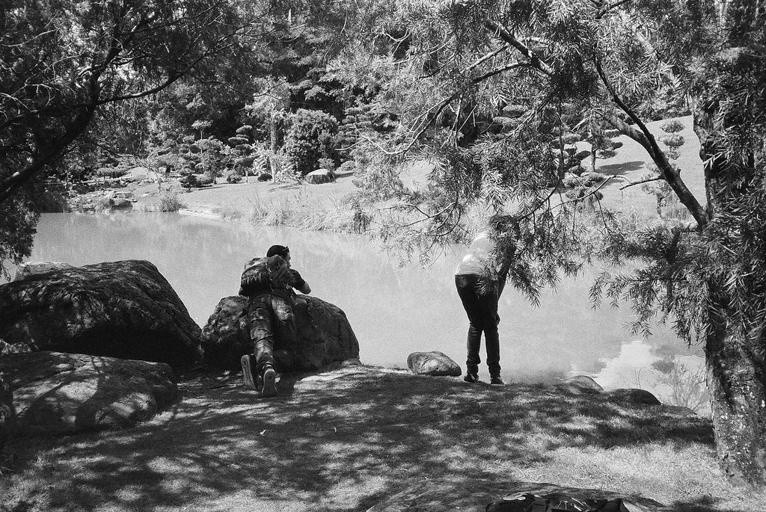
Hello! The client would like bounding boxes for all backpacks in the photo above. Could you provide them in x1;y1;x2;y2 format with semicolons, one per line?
241;254;288;293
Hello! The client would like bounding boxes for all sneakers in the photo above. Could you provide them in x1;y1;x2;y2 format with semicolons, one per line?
259;363;280;398
490;378;505;387
464;373;479;382
240;353;258;393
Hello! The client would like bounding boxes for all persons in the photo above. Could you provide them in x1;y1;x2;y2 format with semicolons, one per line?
452;214;520;385
238;244;311;400
